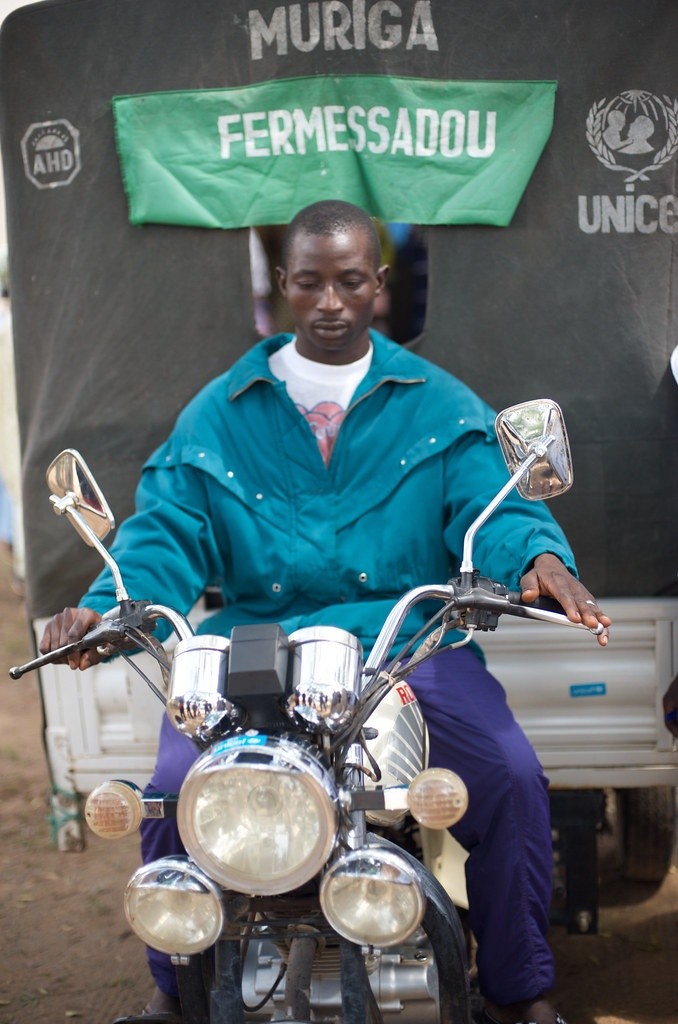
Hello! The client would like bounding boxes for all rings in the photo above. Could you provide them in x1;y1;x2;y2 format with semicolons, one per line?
586;600;595;605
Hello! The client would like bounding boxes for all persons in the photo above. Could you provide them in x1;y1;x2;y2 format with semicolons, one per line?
39;200;611;1024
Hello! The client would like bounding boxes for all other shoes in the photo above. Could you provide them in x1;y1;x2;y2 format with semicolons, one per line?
481;1002;566;1024
141;1003;178;1017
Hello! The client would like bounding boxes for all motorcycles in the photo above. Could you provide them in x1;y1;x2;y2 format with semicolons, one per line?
6;397;606;1024
